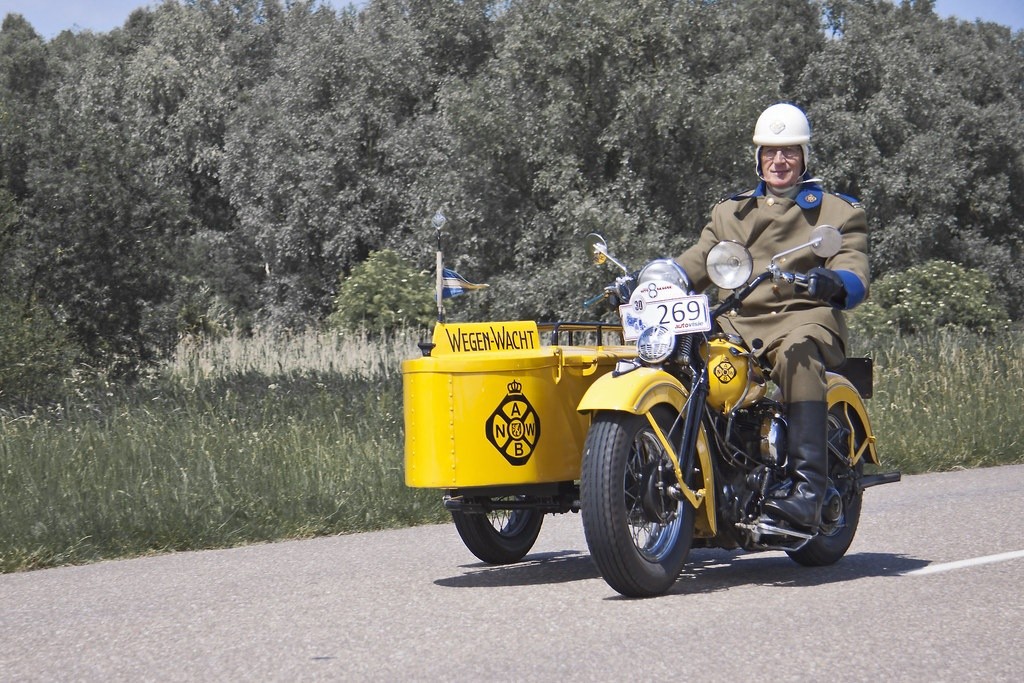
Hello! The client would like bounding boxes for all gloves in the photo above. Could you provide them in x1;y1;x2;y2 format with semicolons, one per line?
594;270;640;316
795;267;843;303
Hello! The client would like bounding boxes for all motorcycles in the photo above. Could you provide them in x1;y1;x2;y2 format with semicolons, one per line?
400;224;901;598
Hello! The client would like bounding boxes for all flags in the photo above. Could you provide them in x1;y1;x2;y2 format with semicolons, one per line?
440;259;490;301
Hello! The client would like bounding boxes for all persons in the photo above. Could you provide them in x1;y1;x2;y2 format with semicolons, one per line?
629;103;868;535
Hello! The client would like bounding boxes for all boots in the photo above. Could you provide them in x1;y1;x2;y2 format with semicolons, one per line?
763;401;828;534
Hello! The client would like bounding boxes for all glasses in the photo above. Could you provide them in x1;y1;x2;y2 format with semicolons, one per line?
759;147;801;158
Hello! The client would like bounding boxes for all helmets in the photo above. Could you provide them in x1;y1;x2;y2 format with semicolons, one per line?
752;103;811;146
